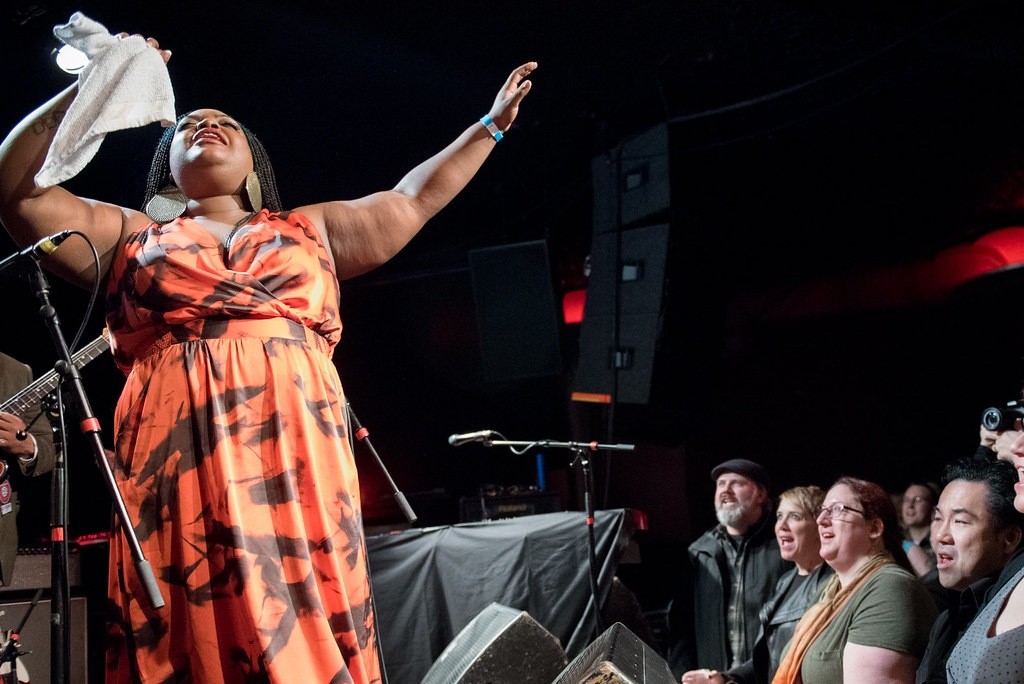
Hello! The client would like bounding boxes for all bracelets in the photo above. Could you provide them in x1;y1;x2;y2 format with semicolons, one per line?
478;115;505;144
900;535;915;553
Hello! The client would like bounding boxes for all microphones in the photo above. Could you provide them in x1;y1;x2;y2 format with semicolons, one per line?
0;228;72;281
448;428;492;447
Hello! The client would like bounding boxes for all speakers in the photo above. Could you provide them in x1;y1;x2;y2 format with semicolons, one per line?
550;620;678;684
464;234;567;384
1;588;89;684
416;602;571;683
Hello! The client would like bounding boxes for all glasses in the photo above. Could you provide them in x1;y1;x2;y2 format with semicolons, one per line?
902;495;929;505
815;504;865;518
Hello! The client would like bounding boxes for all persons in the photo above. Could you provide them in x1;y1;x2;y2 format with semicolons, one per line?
595;524;657;649
773;475;937;684
945;403;1024;684
678;457;795;684
679;484;835;684
882;476;962;613
911;453;1024;684
539;470;579;512
0;350;59;590
0;33;541;684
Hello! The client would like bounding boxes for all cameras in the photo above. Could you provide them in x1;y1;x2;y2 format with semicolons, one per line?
980;398;1024;434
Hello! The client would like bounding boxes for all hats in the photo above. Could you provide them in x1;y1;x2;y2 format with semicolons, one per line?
711;459;766;486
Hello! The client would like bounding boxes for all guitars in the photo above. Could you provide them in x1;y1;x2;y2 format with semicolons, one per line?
0;327;111;442
